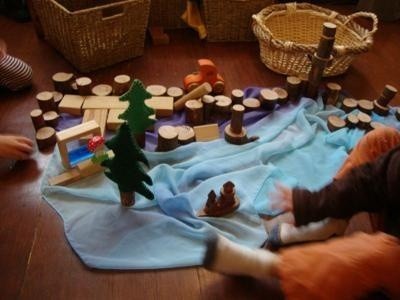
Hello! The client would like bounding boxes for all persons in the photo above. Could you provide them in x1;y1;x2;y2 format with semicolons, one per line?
1;44;34;160
203;128;399;300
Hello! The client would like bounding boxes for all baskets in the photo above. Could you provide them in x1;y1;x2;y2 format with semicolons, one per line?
201;0;279;45
28;0;151;73
251;2;378;78
147;0;191;30
